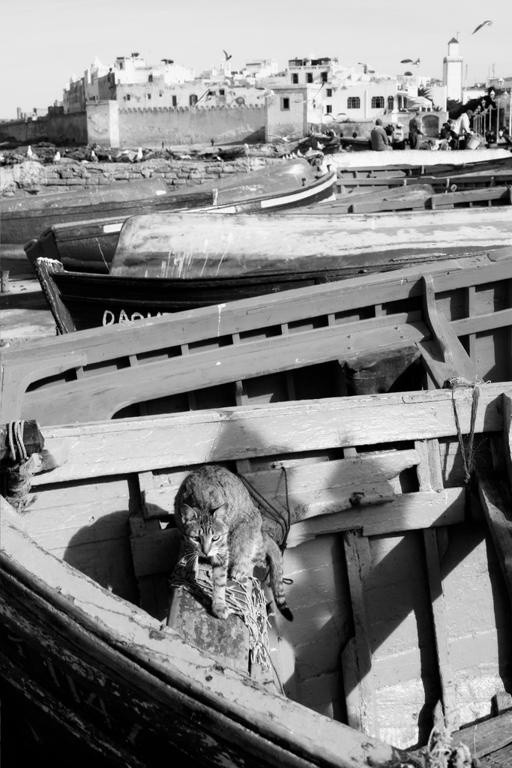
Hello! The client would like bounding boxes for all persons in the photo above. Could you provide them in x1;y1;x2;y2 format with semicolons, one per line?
323;109;511;155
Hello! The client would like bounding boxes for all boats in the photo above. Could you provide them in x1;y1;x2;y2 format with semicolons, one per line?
0;151;340;300
328;146;511;185
0;247;511;431
24;203;512;332
0;380;512;768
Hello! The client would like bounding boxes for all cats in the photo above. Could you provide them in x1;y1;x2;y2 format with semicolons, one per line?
172;463;295;622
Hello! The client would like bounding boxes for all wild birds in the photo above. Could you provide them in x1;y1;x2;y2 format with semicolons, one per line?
90;150;99;162
26;144;34;158
401;58;422;65
357;62;375;75
132;147;143;162
282;141;327;161
222;48;232;61
472;19;492;34
161;58;174;65
52;150;62;165
